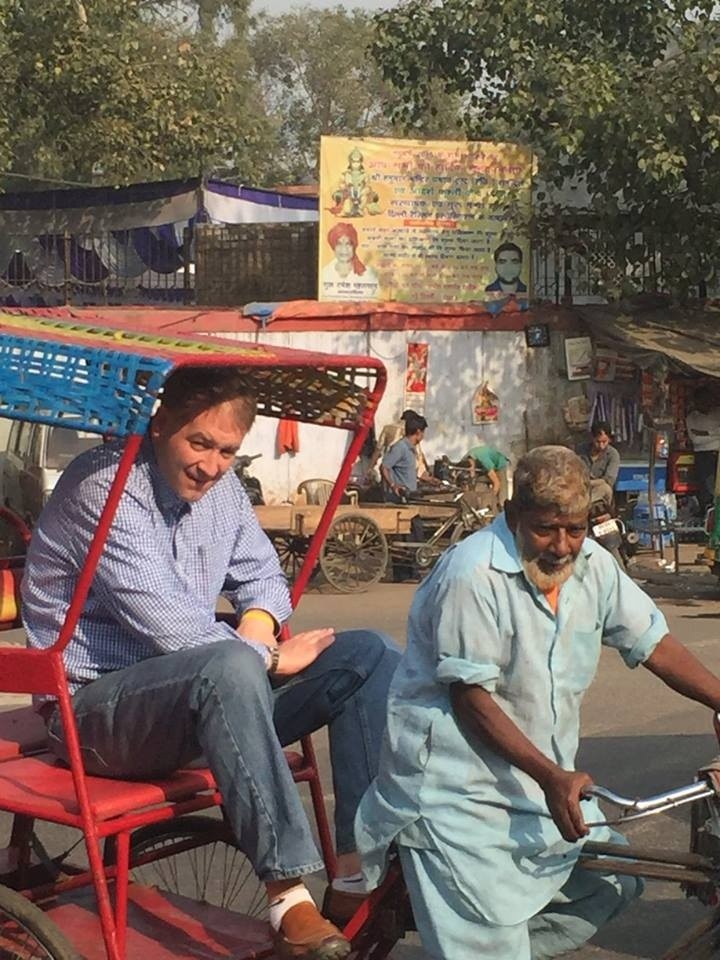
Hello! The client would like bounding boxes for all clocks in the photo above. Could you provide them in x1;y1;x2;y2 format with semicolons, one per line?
525;323;551;347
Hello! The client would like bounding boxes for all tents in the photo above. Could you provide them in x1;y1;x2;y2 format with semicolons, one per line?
0;178;317;308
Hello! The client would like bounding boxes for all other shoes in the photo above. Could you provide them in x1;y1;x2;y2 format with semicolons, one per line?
389;556;423;585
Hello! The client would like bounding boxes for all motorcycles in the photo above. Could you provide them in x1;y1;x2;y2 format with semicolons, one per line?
585;479;639;575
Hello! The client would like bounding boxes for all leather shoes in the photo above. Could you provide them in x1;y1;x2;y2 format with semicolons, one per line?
269;900;352;960
322;887;372;924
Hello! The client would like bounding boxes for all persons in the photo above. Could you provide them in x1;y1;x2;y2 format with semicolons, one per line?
17;367;415;958
455;446;511;512
317;222;381;300
576;422;621;507
353;439;717;959
485;242;527;294
380;414;431;583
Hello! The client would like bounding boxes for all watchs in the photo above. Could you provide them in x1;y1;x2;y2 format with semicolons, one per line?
265;643;279;674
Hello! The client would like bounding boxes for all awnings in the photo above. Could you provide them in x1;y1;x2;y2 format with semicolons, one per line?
576;298;720;378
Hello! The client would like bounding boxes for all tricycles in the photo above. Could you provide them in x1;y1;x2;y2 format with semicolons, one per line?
1;302;720;960
234;477;500;597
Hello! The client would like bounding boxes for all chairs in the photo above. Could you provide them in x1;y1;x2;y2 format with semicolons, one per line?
1;554;337;958
627;504;680;575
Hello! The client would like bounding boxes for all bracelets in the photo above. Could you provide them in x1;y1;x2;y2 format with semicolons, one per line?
390;482;395;488
239;612;276;631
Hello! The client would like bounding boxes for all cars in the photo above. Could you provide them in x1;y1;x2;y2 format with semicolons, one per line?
0;406;106;522
